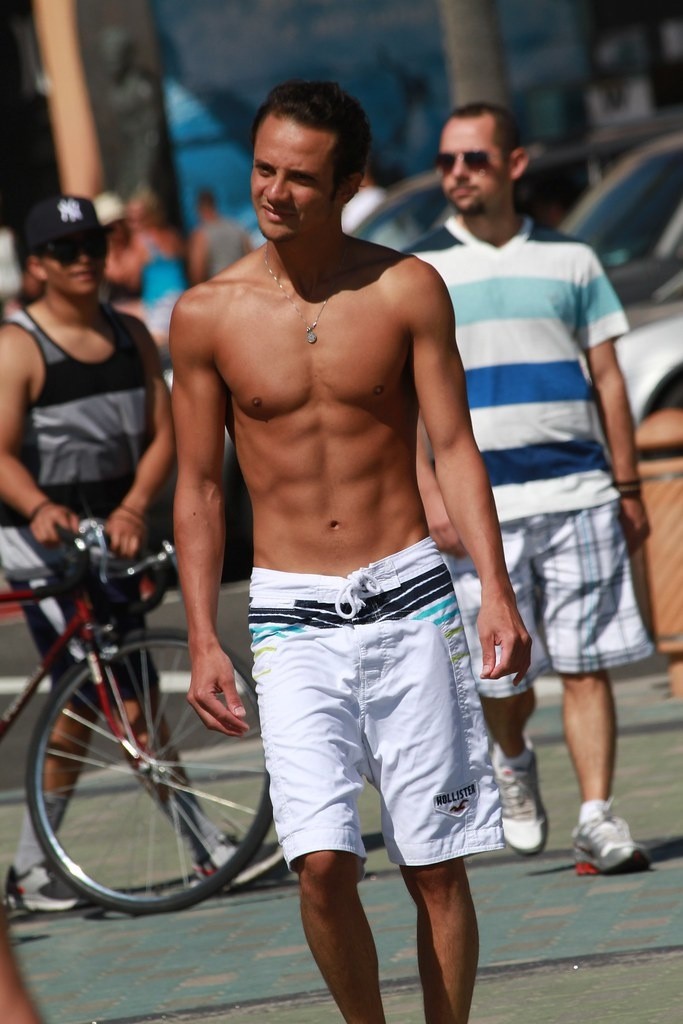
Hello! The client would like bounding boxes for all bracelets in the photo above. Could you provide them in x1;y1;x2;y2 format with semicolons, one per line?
27;498;51;523
614;479;641;497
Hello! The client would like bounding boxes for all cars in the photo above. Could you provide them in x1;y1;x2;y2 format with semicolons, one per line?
344;114;683;265
545;130;683;472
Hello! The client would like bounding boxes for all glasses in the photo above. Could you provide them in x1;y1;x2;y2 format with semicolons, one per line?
435;151;508;176
41;234;109;266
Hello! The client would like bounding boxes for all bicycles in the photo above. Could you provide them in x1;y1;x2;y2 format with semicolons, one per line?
1;522;277;915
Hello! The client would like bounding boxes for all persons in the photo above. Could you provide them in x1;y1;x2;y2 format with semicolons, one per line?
340;147;388;234
519;164;578;234
0;192;286;915
89;185;255;394
399;102;657;876
166;81;535;1024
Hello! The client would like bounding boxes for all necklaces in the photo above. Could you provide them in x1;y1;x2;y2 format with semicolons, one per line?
264;232;352;343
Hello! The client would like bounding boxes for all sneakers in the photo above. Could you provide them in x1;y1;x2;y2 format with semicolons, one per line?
572;796;650;876
492;737;548;855
191;833;284;892
4;862;89;911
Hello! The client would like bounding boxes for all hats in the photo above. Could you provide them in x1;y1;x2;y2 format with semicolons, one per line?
27;196;116;248
